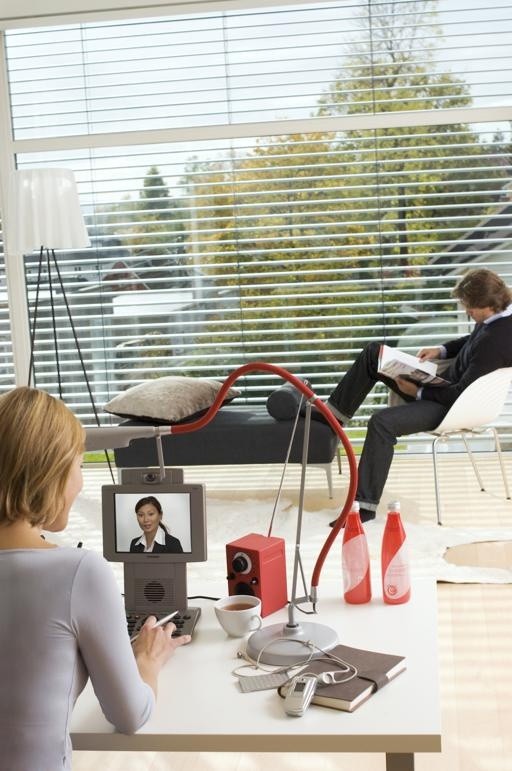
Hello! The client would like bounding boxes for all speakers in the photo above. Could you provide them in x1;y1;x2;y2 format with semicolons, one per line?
123;562;187;614
225;533;287;618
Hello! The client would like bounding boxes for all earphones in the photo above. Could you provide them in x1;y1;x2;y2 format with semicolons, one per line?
237;651;244;658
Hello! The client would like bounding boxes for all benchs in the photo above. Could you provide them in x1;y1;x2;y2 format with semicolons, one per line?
106;406;345;501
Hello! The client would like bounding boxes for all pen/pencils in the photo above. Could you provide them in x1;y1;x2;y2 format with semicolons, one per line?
130;610;179;644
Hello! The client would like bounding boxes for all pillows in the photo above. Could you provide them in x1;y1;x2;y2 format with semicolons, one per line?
101;375;314;425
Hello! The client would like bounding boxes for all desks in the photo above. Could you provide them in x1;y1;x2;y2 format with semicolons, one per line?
71;577;444;771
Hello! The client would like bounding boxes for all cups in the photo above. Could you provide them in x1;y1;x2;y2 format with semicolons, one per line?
213;595;266;640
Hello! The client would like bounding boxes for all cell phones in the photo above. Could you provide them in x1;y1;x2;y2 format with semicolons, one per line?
283;675;318;717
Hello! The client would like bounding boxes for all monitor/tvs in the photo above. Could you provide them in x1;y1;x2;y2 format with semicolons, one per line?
102;484;208;562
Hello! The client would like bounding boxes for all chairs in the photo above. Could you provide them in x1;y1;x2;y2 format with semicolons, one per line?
383;360;511;525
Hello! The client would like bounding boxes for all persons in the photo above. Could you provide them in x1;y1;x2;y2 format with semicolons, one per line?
128;495;186;554
1;386;192;771
300;267;511;528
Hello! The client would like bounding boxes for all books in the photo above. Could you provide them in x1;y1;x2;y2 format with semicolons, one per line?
377;343;452;388
277;642;408;714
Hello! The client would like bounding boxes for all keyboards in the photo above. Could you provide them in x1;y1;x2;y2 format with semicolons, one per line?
126;607;202;644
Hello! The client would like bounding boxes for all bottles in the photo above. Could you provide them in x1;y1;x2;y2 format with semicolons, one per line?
340;501;412;605
